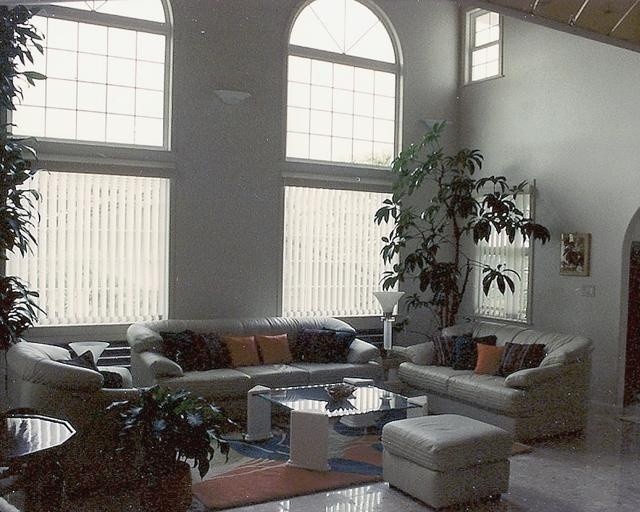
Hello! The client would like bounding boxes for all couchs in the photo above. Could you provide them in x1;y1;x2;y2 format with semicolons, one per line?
6;342;138;432
398;322;594;443
127;317;381;400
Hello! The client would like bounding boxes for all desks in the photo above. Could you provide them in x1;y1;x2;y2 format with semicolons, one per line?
0;414;77;512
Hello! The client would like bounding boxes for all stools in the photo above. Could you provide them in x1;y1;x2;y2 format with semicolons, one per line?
381;414;513;510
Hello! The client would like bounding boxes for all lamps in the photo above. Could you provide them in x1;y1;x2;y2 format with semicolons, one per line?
373;292;405;381
68;343;109;368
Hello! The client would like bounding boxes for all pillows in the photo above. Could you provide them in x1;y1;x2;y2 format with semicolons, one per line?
159;328;355;370
55;350;122;388
433;333;546;377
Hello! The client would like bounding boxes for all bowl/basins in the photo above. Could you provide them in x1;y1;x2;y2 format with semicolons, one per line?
324;384;357;401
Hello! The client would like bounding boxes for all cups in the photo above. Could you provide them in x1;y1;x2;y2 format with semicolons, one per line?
68;341;110;366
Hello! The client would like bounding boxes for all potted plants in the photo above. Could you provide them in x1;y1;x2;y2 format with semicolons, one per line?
103;386;245;512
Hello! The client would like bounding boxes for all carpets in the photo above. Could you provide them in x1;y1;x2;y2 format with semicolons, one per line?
178;421;533;509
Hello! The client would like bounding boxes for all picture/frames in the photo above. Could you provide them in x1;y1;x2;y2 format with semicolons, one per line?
561;232;589;276
